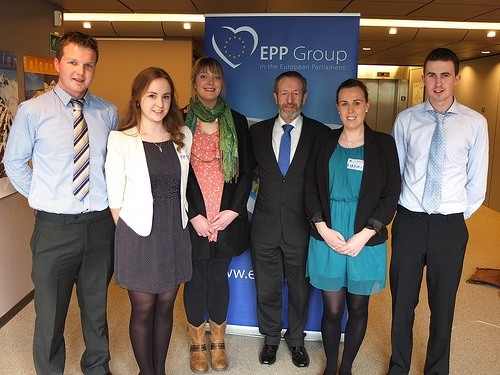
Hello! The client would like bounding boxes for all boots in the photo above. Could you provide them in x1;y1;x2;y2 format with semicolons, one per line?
208;319;229;371
187;319;209;374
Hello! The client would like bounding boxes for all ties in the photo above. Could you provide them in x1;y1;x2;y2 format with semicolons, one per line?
278;124;294;177
70;98;90;201
422;113;446;215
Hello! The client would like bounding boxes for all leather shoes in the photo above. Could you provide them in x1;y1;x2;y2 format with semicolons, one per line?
288;345;310;368
259;344;279;365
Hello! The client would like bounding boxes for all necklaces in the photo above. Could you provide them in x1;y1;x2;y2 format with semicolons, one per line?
141;126;164;152
346;139;354;148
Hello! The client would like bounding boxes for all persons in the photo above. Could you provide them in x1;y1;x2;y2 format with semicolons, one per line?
386;47;489;375
3;32;118;375
305;78;401;375
248;71;332;368
104;67;193;375
178;57;248;374
